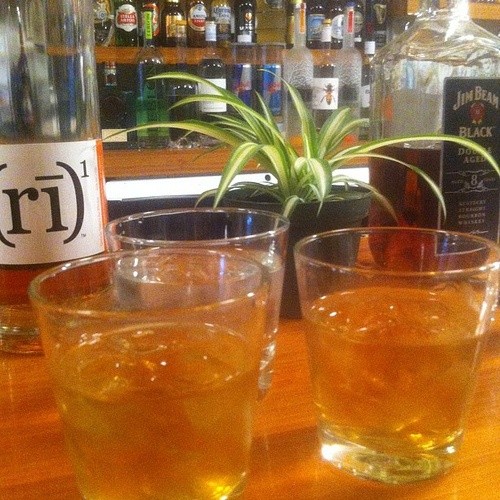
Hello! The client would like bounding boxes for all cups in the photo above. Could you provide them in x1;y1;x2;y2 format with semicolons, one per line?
293;225;500;486
105;207;291;402
28;247;272;499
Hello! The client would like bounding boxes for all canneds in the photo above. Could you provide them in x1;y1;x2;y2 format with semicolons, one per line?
231;63;252;113
261;63;283;115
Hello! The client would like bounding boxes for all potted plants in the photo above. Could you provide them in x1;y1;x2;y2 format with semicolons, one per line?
101;65;500;320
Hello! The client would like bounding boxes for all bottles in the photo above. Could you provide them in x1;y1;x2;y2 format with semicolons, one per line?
369;0;500;279
93;0;395;150
0;0;120;355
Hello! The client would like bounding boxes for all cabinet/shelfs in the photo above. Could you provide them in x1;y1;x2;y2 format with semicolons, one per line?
46;0;500;180
